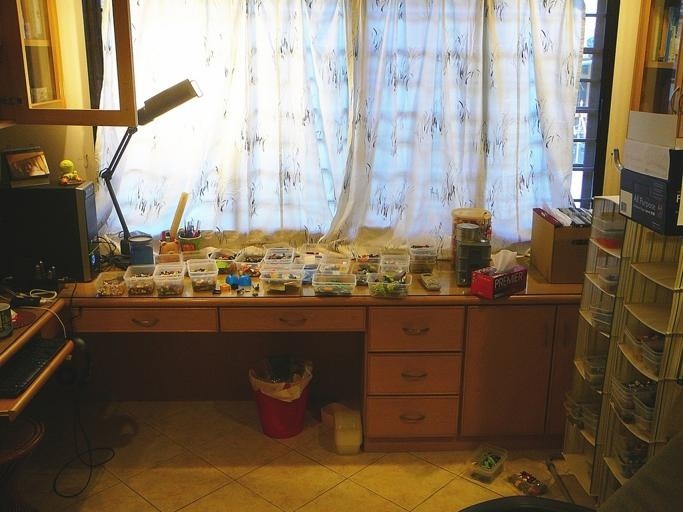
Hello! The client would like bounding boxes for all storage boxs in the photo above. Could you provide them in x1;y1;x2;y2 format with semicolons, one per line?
616;106;683;235
528;209;591;285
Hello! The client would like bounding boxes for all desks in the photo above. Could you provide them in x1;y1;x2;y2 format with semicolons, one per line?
1;251;584;454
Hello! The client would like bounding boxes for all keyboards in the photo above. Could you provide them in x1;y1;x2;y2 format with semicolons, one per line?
0;338;66;398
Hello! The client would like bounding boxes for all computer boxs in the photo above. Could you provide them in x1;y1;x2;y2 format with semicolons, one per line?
0;177;100;283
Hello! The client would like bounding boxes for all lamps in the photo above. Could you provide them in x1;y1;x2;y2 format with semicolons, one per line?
99;77;205;272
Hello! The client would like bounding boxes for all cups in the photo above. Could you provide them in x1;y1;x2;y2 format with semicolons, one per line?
0;302;11;338
158;233;201;254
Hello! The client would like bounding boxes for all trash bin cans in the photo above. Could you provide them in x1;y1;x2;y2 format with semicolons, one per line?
247;363;312;438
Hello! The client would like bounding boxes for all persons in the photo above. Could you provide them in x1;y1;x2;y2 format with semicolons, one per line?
11;161;39;177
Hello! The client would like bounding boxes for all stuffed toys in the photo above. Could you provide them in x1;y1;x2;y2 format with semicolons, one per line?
58;160;82;186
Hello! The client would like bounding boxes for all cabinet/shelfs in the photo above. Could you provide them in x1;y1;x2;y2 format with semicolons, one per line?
624;1;683;139
0;0;139;131
544;194;682;510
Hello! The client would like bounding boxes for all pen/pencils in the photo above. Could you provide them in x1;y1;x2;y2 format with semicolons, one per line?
184;219;201;238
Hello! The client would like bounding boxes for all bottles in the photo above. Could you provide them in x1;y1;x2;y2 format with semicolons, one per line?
451;207;490;286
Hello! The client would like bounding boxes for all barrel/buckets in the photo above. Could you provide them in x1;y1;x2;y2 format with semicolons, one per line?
456;241;492;287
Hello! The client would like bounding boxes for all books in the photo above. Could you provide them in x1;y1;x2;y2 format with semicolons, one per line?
650;0;683;115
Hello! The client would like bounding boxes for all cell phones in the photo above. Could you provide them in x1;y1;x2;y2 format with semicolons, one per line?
11;295;41;306
